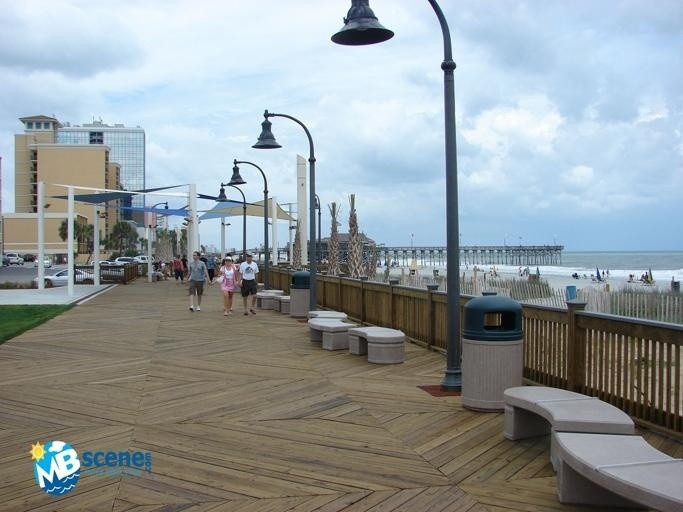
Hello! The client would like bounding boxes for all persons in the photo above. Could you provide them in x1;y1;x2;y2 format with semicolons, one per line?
185;250;211;311
465;262;541;280
210;256;240;316
152;253;234;282
570;265;656;285
238;253;259;315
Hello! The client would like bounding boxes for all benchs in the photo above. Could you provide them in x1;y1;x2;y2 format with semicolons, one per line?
256;289;290;314
348;326;406;364
307;310;358;351
502;386;683;507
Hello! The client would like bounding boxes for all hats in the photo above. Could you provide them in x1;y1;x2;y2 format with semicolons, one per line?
225;256;231;261
246;254;252;257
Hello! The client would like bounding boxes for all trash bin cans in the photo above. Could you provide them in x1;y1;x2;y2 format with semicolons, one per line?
290;271;310;318
461;292;524;413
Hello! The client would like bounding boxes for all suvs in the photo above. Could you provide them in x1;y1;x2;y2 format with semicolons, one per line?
5;253;24;265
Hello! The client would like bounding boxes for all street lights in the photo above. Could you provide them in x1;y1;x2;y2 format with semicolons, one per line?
331;1;460;393
214;183;246;262
251;110;317;323
313;194;322;264
225;159;269;290
147;202;172;283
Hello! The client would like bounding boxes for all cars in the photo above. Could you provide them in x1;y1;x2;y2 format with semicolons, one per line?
32;255;51;268
78;248;155;283
31;267;102;288
2;252;9;266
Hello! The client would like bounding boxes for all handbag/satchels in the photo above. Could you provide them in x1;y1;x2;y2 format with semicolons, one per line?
216;266;225;284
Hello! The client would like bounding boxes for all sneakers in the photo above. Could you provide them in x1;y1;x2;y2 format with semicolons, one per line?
189;306;256;317
